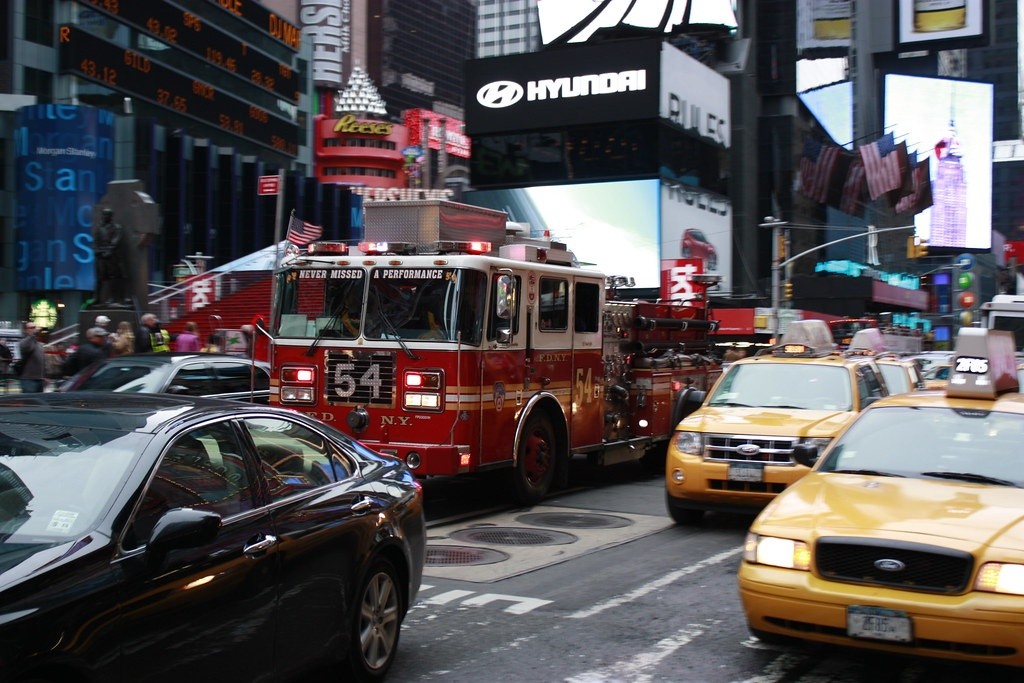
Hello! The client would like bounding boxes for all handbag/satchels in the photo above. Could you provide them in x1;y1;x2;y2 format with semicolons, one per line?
12;346;35;377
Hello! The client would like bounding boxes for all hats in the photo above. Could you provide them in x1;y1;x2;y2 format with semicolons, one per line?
86;327;110;338
96;315;111;324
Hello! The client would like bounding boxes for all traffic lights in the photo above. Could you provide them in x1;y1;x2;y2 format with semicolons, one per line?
914;236;927;257
785;283;791;299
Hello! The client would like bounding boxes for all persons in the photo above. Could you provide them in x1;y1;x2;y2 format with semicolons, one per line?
342;278;473;342
92;208;124;304
0;314;252;393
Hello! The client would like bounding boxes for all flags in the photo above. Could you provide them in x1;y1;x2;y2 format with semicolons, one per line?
799;133;935;219
287;214;324;245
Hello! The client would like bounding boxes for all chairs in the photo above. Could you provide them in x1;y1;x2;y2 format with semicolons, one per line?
167;437;218;477
258;443;324;500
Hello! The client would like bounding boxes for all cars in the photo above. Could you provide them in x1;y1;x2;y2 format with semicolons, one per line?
735;327;1024;670
54;355;271;410
0;390;425;683
843;328;1024;439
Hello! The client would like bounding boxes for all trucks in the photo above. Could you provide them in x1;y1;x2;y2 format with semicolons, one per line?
982;296;1024;362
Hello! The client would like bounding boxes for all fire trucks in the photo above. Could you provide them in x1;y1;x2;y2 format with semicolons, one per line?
255;195;726;502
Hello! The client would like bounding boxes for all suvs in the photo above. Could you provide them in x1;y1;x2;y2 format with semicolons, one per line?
665;319;896;523
